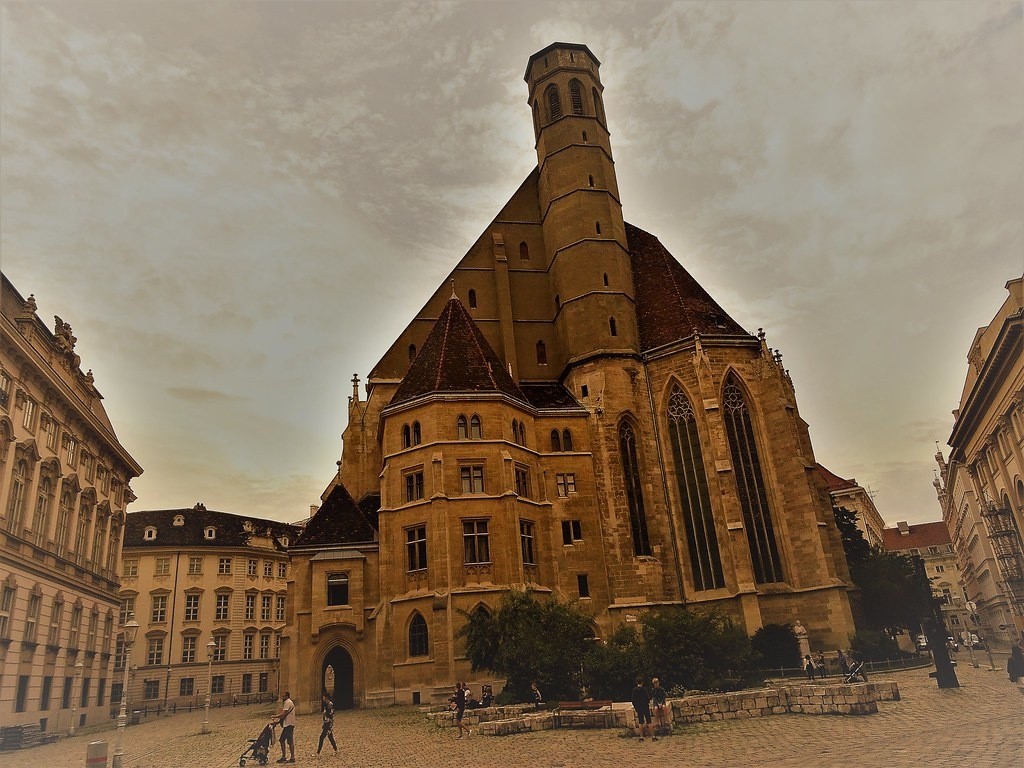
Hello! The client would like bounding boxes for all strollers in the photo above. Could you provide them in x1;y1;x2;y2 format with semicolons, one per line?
239;720;276;767
841;661;864;684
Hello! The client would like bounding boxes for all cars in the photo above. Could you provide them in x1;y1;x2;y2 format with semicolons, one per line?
917;635;959;654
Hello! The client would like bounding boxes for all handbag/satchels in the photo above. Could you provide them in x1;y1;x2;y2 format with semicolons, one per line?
806;661;815;675
818;663;825;668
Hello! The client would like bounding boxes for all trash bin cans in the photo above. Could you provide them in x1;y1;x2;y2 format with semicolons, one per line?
413;691;420;705
482;685;492;699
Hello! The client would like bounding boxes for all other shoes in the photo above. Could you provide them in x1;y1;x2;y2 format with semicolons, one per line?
276;757;287;762
468;729;472;736
286;758;295;763
455;737;462;739
639;738;644;742
652;738;658;742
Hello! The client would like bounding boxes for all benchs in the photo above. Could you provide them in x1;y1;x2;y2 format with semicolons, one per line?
553;700;613;728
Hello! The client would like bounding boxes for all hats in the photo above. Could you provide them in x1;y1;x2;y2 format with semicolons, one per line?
652;677;658;682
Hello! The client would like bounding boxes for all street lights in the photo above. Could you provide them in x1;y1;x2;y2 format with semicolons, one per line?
127;662;137;723
952;591;979;668
102;612;140;768
67;657;82;736
201;633;216;734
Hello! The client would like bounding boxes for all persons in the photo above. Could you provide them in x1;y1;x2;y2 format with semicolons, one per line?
652;678;668;725
311;692;339;757
270;691;296;762
804;649;859;681
455;682;489;740
632;677;659;742
793;620;807;634
1008;647;1024;695
532;684;541;713
